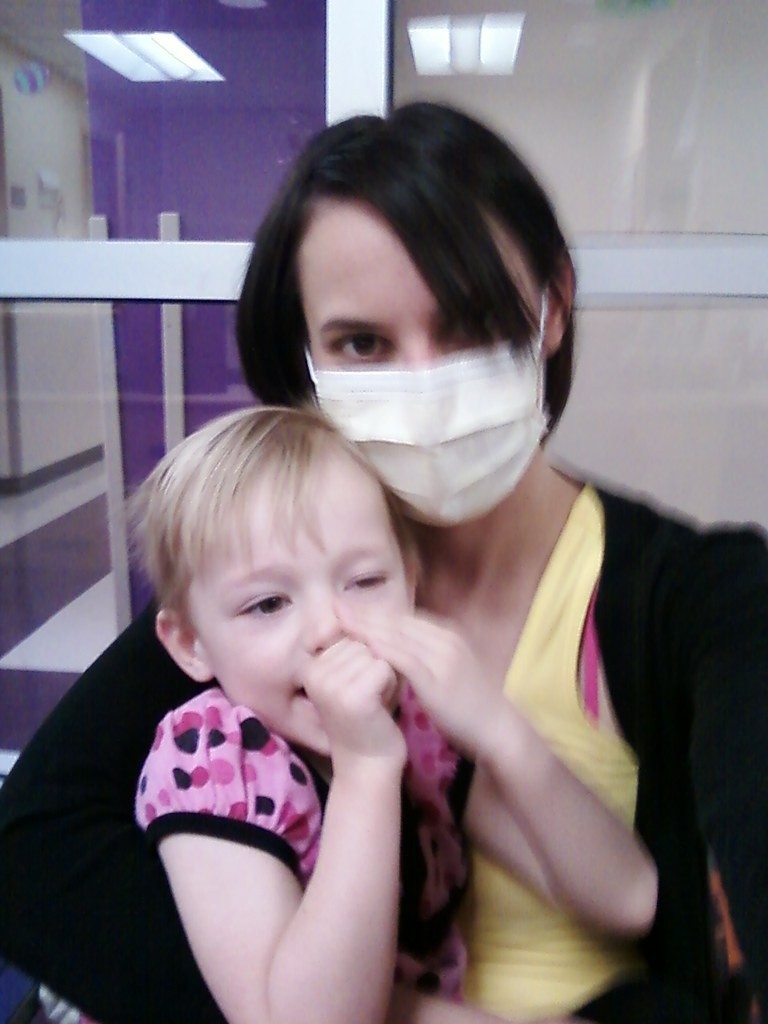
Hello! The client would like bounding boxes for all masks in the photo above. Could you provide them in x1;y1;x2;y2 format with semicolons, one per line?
298;286;549;530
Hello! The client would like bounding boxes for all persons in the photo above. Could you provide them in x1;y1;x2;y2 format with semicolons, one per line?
117;403;685;1024
1;97;768;1024
